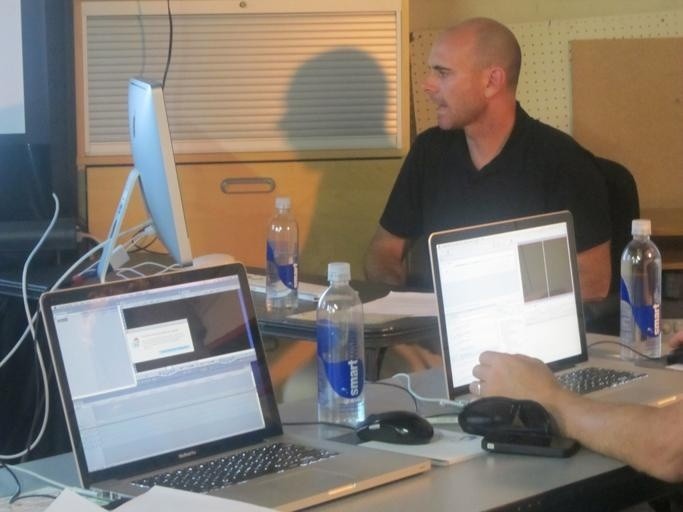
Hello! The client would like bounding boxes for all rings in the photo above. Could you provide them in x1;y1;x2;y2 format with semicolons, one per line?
477;383;480;395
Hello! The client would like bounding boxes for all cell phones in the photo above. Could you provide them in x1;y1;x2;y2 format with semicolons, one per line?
480;429;579;460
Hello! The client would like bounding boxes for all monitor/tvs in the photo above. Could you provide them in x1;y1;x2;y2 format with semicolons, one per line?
97;78;193;282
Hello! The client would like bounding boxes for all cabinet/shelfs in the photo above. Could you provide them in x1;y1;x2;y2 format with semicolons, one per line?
69;3;417;281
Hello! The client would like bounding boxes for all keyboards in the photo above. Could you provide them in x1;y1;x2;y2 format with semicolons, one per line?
250;274;338;302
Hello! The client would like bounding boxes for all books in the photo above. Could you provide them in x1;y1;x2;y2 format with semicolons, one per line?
356;426;490;465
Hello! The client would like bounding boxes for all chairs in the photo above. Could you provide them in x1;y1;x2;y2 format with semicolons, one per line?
582;154;644;324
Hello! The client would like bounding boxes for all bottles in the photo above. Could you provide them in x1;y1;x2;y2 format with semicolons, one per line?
618;219;663;359
266;195;298;312
315;260;364;428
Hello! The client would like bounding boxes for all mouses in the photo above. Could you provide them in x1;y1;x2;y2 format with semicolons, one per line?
358;411;432;446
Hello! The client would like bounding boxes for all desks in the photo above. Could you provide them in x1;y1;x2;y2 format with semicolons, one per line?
0;333;683;510
634;207;682;274
0;248;439;457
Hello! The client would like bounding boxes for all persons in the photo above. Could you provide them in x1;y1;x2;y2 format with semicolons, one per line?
283;18;613;406
468;351;683;483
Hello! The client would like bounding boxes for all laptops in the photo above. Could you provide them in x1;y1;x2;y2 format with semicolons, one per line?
38;264;429;512
428;210;683;405
